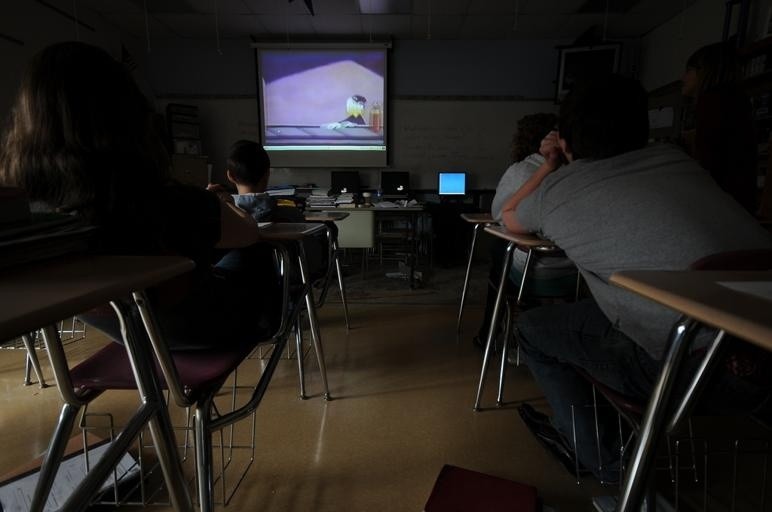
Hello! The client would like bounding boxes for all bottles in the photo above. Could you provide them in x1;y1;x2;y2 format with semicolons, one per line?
363;192;372;207
367;99;380;134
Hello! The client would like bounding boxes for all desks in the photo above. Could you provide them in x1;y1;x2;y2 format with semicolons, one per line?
268;201;427;291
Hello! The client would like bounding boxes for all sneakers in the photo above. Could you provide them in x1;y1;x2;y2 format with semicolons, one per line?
473;335;496;360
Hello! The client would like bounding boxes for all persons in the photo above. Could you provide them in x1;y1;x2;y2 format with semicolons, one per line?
0;41;283;355
474;113;563;350
681;40;757;213
226;140;276;221
501;81;772;406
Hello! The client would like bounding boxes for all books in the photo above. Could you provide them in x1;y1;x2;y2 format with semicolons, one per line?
266;187;294;196
306;186;336;208
0;431;141;512
424;465;540;511
741;55;766;80
336;192;356;209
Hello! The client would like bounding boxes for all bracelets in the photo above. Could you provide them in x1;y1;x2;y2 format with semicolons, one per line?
211;189;221;192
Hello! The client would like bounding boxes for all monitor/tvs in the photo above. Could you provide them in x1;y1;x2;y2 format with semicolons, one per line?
437;171;468;196
379;171;410;199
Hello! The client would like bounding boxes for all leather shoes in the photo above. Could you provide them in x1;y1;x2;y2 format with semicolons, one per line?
517;401;592;479
